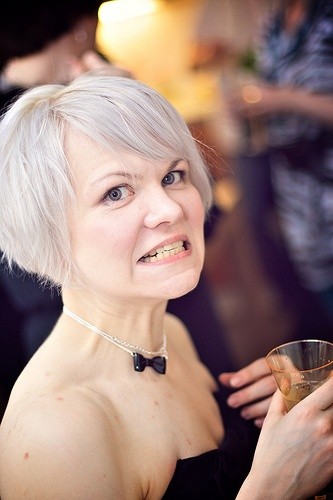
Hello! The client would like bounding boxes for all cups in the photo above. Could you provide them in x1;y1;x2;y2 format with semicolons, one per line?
266;339;333;413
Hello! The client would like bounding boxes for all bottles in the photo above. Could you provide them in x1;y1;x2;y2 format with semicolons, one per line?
232;45;267;156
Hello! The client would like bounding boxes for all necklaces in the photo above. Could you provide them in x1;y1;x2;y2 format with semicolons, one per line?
62;305;168;374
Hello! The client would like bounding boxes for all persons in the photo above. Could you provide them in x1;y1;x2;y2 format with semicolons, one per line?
0;0;237;420
228;0;333;376
0;75;333;500
